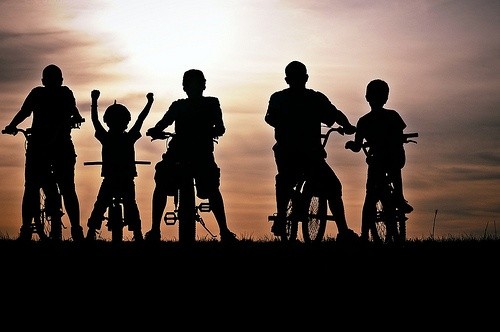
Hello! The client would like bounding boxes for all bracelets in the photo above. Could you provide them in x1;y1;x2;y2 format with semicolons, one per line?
91;105;98;107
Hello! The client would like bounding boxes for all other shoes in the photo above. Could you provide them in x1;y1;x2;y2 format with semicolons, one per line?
221;229;239;242
16;229;32;240
85;231;96;241
359;235;369;241
272;221;282;236
146;229;161;239
392;193;413;213
337;229;359;242
71;226;84;240
135;234;144;243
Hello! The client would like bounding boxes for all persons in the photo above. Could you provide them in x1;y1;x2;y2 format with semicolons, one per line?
346;79;414;242
84;89;154;240
144;70;239;241
265;61;360;242
5;65;84;241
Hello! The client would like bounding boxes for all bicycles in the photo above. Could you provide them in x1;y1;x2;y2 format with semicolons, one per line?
1;119;84;246
145;130;218;248
84;161;152;243
267;126;345;243
344;133;419;244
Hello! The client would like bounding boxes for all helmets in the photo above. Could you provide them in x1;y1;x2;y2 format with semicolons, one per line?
104;104;131;122
